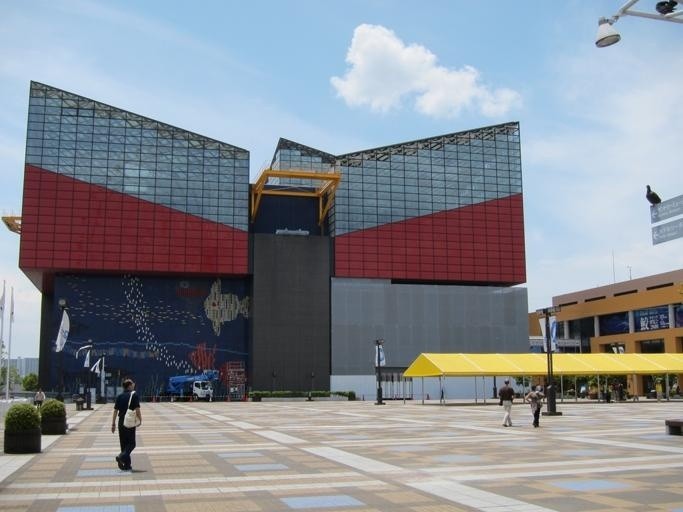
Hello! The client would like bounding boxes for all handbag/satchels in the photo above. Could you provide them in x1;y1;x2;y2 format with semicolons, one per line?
123;408;140;428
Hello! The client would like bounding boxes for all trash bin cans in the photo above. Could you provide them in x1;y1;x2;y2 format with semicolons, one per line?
76;401;82;410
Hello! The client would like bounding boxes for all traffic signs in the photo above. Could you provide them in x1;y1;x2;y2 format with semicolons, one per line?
650;195;683;224
652;218;683;245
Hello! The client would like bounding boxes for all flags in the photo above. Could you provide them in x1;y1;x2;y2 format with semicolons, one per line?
50;306;72;353
75;343;101;373
373;346;387;366
538;316;557;353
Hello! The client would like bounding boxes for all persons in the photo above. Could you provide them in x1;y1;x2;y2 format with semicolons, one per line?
498;378;515;427
110;378;141;470
524;384;545;427
32;386;44;410
575;378;682;403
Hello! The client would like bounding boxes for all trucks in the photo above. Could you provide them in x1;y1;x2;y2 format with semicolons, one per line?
169;375;213;399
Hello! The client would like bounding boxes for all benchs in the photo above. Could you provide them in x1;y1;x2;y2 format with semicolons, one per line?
665;418;683;435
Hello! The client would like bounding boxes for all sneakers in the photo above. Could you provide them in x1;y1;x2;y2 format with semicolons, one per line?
116;456;132;470
503;424;539;427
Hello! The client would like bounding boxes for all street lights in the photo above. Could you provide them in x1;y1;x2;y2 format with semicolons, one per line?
84;337;93;411
375;336;385;405
99;352;107;404
57;298;65;401
536;305;561;415
